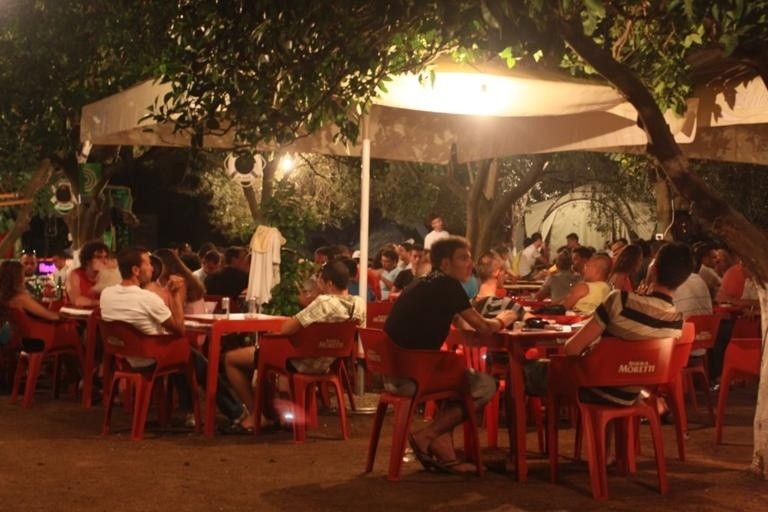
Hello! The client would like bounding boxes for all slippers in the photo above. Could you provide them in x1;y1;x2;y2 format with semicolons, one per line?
405;431;478;477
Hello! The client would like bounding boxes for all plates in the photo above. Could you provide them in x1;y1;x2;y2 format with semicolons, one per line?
526;317;548;328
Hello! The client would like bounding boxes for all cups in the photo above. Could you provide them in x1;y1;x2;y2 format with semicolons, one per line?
506;321;513;330
570;323;581;331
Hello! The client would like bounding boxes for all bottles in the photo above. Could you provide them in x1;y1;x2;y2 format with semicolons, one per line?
33;274;63;301
511;294;516;302
222;297;257;319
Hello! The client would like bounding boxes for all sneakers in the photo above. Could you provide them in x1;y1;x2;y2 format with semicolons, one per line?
184;409;205;429
216;406;272;436
483;458;519;481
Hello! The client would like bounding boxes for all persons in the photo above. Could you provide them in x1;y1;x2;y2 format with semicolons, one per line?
1;219;760;475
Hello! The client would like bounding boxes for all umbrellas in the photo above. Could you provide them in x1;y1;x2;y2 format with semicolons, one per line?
584;70;768;165
80;51;701;395
